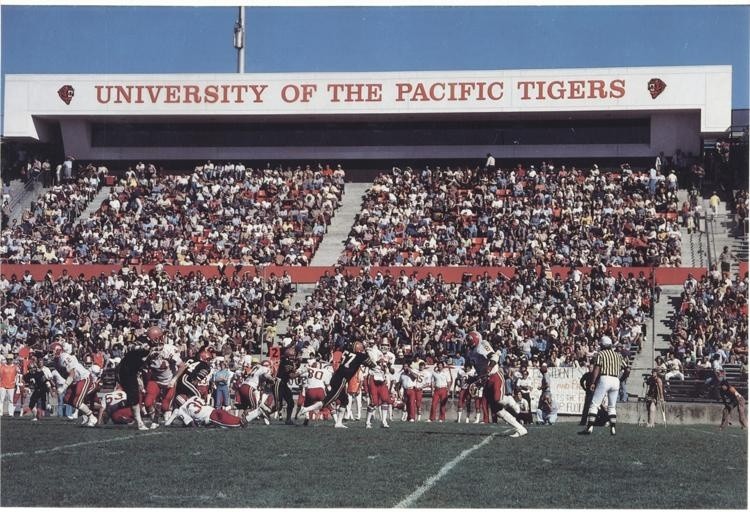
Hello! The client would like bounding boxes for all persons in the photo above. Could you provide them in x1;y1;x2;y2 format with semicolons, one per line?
577;334;621;435
459;331;528;438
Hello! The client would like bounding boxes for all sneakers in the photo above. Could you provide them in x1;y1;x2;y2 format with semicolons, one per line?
66;408;617;438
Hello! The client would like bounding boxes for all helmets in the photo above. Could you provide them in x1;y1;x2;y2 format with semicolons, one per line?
199;350;212;362
600;336;612;349
284;348;296;358
49;343;62;359
379;336;390;352
466;330;482;348
173;393;188;405
147;326;163;341
353;341;364;353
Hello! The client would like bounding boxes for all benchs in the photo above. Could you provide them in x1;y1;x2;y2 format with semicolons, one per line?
663;233;748;404
